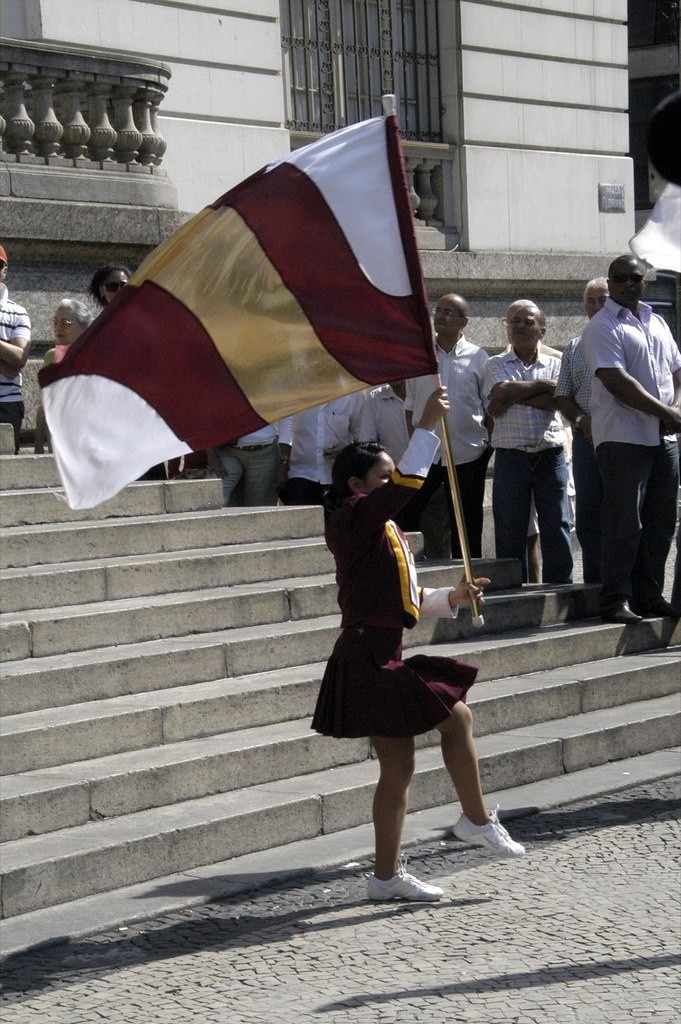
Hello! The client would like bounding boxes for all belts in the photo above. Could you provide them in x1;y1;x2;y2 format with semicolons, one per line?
234;444;271;452
514;444;550;453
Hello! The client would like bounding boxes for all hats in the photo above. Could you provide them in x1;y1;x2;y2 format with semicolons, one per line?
0;245;8;265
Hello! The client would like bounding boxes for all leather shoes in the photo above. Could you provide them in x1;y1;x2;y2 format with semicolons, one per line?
599;603;643;622
630;595;681;617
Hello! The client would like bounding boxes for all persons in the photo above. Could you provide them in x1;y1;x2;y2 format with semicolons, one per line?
167;377;420;533
404;255;681;621
87;266;167;481
0;246;32;452
34;298;95;454
308;387;526;900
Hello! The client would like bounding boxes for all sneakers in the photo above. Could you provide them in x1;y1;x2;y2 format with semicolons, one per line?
364;853;444;900
454;802;525;859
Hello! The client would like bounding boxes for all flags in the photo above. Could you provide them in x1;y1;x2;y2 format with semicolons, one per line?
35;118;443;511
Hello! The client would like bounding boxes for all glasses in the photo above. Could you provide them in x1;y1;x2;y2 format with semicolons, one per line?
101;281;128;292
49;316;79;329
587;295;607;307
610;273;645;284
430;307;455;320
0;261;4;269
501;318;511;328
